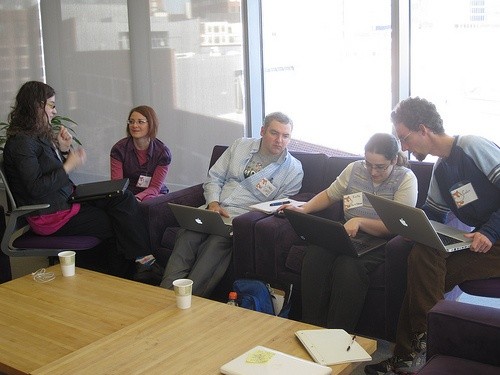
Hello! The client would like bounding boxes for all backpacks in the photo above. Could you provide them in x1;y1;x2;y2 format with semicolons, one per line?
229;267;295;318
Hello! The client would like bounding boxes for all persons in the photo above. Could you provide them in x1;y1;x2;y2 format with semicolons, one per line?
2;80;164;286
110;105;172;203
275;132;418;334
160;112;304;299
364;96;500;375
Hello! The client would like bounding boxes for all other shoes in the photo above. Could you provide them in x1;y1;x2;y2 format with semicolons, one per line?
364;334;438;375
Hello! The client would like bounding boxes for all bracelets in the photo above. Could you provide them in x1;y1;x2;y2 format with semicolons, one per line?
57;148;70;155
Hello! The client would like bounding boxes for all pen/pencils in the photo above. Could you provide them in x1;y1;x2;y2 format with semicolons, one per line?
270;201;290;206
347;335;357;351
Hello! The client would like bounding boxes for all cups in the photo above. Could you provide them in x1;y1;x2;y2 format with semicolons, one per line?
172;278;194;310
58;251;76;276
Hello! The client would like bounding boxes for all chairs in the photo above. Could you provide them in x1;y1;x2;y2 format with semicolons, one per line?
0;160;101;266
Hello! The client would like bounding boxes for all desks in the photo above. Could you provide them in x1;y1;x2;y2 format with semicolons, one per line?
1;264;376;375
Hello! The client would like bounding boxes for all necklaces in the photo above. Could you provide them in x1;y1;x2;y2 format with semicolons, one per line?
372;182;385;195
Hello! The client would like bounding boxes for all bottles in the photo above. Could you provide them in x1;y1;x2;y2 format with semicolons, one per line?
226;292;239;307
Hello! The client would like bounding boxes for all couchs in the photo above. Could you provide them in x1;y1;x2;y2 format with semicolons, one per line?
139;146;500;375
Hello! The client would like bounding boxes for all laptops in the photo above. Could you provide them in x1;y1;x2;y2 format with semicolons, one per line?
282;209;387;257
68;178;129;204
167;202;233;237
365;191;474;251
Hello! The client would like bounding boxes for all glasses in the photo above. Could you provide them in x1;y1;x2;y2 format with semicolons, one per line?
41;101;56;109
361;158;390;170
127;120;149;125
395;131;414;143
